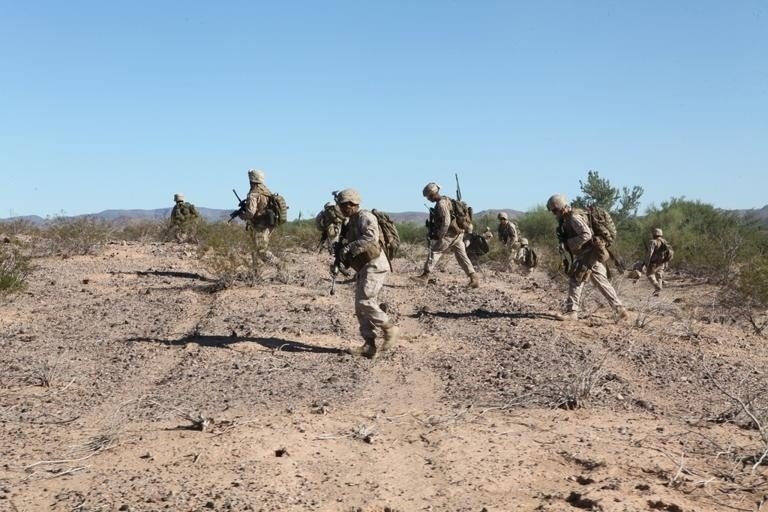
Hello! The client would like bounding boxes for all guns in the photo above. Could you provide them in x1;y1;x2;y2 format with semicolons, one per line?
228;190;248;232
330;223;350;296
426;208;435;264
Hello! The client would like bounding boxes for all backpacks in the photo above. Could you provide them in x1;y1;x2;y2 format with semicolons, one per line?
250;186;287;226
513;222;521;242
372;209;400;259
565;207;617;250
445;196;472;231
656;239;673;262
526;249;536;267
316;211;327;230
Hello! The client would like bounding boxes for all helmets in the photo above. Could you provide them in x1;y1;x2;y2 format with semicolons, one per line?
520;238;528;244
653;229;662;235
498;212;508;220
547;195;566;211
338;189;361;205
249;170;264;183
174;194;184;202
324;202;335;207
486;227;489;230
423;183;438;197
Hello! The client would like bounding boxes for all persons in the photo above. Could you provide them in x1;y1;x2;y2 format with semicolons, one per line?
239;170;280;265
325;183;529;358
548;195;629;323
171;194;199;223
646;229;668;296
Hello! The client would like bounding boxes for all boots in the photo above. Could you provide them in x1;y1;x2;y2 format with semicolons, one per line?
614;305;629;323
383;326;399;350
468;274;479;288
347;339;376;357
555;311;578;321
411;271;429;286
276;262;289;284
653;283;662;296
